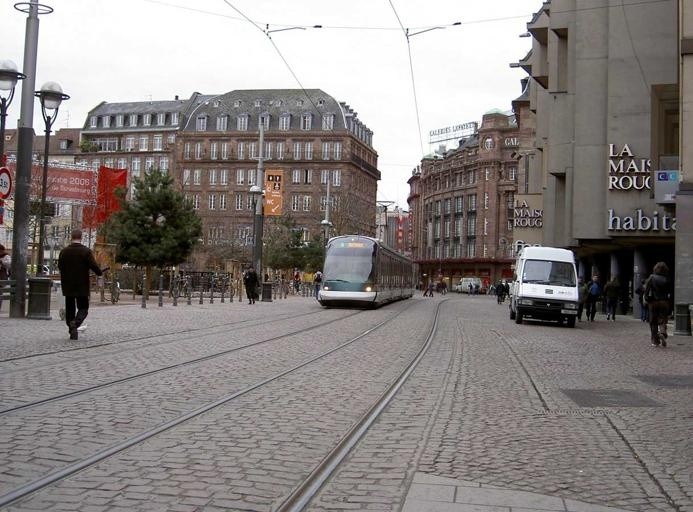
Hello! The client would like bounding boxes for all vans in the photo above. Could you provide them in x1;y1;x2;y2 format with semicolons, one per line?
455;278;481;294
508;246;579;328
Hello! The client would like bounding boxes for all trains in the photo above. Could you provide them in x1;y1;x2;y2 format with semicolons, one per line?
316;234;416;310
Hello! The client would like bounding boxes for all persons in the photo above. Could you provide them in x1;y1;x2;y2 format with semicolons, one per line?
418;277;448;297
294;271;302;294
467;278;510;304
0;245;11;309
243;266;260;304
586;275;602;322
312;272;322;299
577;276;589;322
645;261;673;347
58;229;103;340
636;279;650;321
603;277;623;322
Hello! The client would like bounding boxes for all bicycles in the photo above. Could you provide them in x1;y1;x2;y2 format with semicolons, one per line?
171;277;194;298
104;277;121;304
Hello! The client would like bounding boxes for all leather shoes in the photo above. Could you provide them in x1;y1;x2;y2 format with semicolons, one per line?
69;321;79;340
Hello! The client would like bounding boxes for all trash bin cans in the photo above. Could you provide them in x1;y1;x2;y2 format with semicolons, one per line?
26;278;52;320
261;282;272;302
673;303;692;335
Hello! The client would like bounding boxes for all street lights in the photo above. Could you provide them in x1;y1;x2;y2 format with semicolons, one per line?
25;82;71;276
248;185;263;272
0;59;28;164
320;219;329;263
